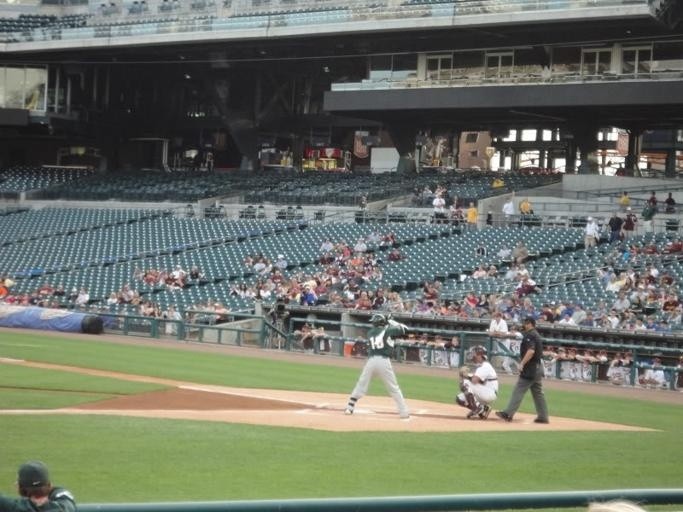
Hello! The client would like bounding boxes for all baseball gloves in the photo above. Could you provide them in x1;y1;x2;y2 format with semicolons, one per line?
459;366;471;378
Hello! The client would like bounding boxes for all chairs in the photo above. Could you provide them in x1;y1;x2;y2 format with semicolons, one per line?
0;207;683;334
0;163;563;207
1;14;93;43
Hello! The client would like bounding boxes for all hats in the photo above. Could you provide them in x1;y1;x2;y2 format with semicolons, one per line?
652;358;661;363
409;333;441;340
521;315;536;327
17;460;50;485
493;313;517;330
615;352;632;357
546;345;607;356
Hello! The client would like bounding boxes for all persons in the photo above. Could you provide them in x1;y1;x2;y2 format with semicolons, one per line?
133;265;201;290
502;190;532;229
0;458;77;512
230;233;411;362
454;344;499;421
107;285;226;334
1;276;90;310
583;191;683;387
471;240;542;297
616;163;625;176
337;312;412;420
413;281;606;382
494;316;550;424
416;183;477;226
512;167;550;176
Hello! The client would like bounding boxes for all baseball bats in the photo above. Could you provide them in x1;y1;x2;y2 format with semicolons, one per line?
373;316;395;347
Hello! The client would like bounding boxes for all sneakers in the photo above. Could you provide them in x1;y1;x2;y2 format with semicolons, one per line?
467;404;492;420
534;418;549;424
495;411;512;422
400;416;411;422
344;408;354;415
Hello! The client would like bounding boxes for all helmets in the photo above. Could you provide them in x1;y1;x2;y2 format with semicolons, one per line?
368;314;385;323
467;345;488;360
